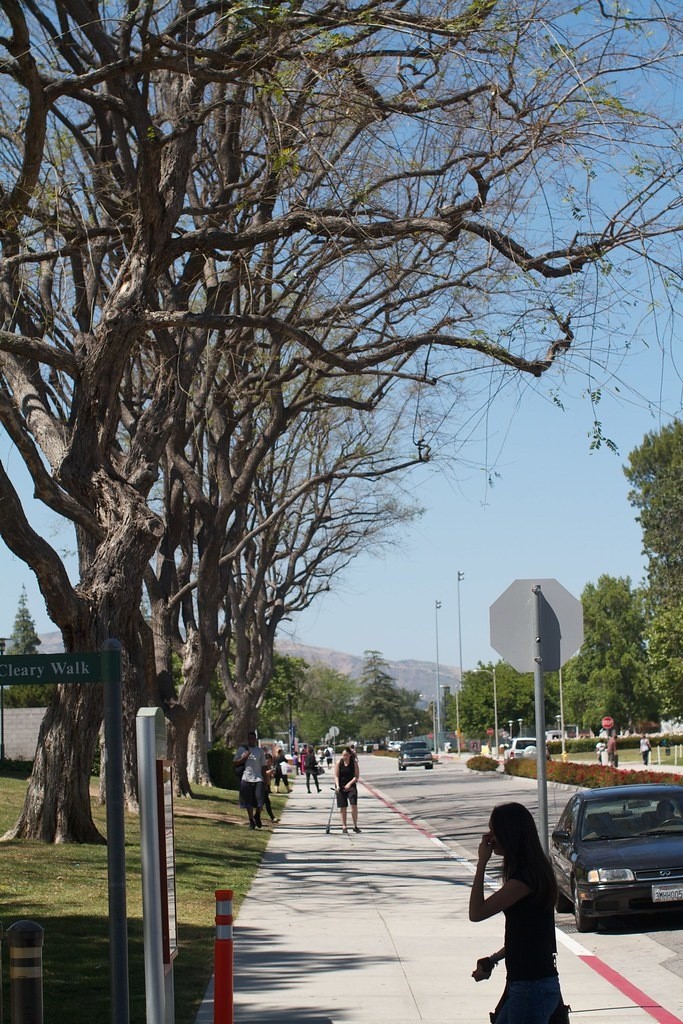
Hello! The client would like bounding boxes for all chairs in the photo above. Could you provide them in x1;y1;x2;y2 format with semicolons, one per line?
586;812;614;836
641;811;660;830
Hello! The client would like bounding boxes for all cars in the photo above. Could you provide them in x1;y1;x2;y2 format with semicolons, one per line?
550;782;682;934
387;741;405;753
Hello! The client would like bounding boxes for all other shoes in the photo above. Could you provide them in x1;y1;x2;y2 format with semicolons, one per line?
353;827;361;833
250;822;255;830
342;826;347;833
272;817;281;823
254;815;262;828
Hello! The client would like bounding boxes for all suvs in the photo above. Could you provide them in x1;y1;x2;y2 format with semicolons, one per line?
398;741;435;770
503;737;549;763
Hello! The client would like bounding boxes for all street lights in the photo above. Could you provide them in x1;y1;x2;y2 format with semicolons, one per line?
472;667;500;761
288;692;294;732
456;570;464;759
434;599;442;732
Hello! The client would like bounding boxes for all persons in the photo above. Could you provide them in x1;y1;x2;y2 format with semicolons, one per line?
468;802;570;1024
233;731;362;833
655;800;680;828
449;732;670;768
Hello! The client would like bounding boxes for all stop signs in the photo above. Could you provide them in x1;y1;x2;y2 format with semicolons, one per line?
602;716;613;729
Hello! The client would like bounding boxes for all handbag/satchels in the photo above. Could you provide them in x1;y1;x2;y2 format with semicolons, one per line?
234;745;249;777
315;764;325;776
648;745;651;750
324;750;330;756
489;981;572;1024
279;761;292;775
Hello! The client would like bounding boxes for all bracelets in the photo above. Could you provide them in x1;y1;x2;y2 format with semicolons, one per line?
494;952;498;964
